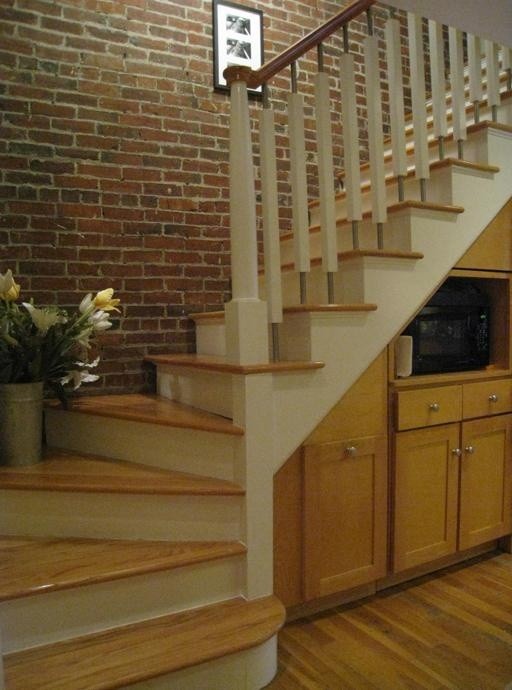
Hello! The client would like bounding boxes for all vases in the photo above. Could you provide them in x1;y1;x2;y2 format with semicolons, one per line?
1;380;49;469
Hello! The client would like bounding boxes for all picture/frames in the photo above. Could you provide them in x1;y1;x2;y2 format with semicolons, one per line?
210;0;266;97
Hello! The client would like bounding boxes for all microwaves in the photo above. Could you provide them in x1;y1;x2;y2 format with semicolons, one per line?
407;305;491;375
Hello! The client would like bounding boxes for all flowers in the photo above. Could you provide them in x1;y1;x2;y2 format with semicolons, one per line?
0;269;121;409
